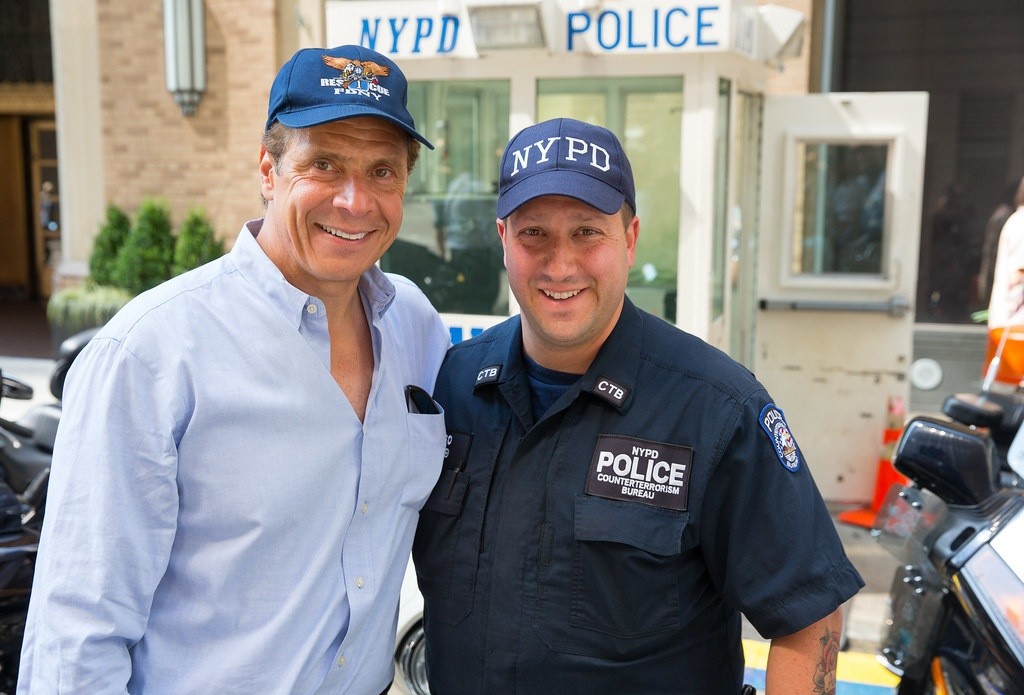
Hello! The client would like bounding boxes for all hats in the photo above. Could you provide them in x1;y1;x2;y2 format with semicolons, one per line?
497;118;636;220
265;45;434;150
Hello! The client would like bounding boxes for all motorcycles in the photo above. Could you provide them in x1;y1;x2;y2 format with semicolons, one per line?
0;326;118;695
873;377;1024;695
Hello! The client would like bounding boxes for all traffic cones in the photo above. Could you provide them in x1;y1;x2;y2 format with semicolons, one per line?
837;396;916;532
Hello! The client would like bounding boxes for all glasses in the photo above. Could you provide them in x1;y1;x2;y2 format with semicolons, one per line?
407;384;440;414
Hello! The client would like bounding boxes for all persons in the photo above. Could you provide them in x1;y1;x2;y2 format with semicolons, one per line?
17;46;451;693
37;179;57;264
984;178;1024;393
413;119;844;695
